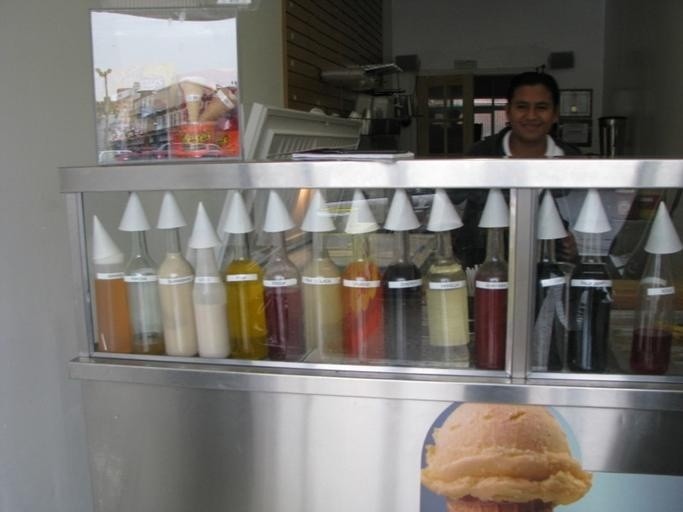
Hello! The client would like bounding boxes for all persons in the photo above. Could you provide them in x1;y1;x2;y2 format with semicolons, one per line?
447;71;585;268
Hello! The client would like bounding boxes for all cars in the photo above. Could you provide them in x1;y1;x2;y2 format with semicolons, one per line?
98;142;223;163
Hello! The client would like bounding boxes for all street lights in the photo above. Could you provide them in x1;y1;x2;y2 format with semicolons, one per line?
95;67;112;151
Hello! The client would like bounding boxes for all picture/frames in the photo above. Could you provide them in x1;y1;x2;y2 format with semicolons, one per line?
557;89;593;117
559;119;592;147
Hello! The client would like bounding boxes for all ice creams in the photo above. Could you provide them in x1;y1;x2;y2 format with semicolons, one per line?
179;76;238;122
420;403;592;511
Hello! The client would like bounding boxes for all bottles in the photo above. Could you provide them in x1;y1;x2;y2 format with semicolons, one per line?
384;230;423;360
93;254;131;354
421;231;470;363
192;243;230;358
568;233;613;372
156;227;198;356
226;232;267;360
340;233;386;360
474;228;510;370
299;232;343;364
262;227;305;360
631;252;676;373
535;238;566;371
126;227;163;355
